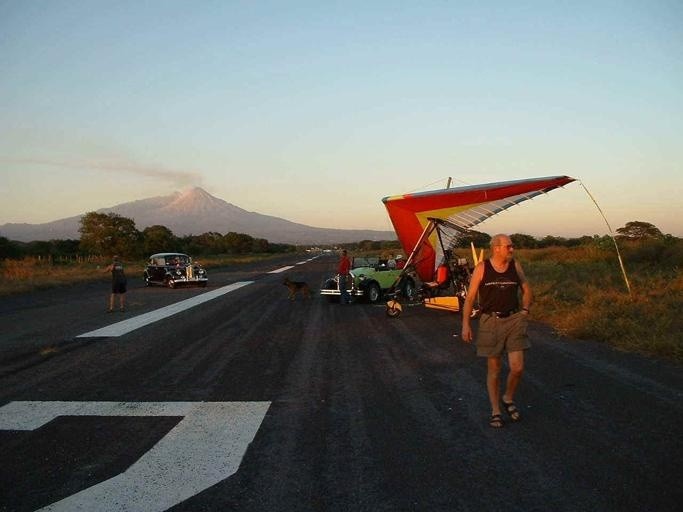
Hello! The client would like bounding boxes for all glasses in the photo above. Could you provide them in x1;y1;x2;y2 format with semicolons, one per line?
494;244;512;249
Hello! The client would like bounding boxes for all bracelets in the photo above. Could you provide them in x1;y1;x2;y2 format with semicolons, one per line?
522;307;529;314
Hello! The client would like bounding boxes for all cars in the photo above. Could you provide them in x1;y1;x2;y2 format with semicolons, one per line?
320;255;415;304
143;252;208;289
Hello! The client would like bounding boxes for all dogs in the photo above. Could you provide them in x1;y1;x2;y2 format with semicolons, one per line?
281;277;312;301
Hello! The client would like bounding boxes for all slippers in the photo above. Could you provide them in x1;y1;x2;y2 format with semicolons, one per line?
503;395;521;422
488;414;505;428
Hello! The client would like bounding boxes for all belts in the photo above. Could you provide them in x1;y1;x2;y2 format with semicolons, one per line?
489;308;519;319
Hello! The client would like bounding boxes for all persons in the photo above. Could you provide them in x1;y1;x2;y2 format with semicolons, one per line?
336;250;354;306
103;254;127;312
387;254;405;269
461;233;532;429
